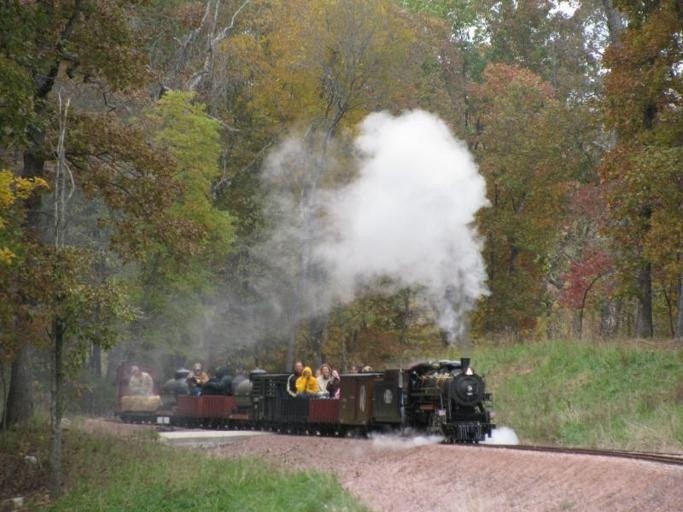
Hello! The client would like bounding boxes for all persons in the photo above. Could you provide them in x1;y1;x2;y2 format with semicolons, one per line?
128;360;372;398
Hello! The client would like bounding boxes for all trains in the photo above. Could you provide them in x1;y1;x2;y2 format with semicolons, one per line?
111;357;495;444
